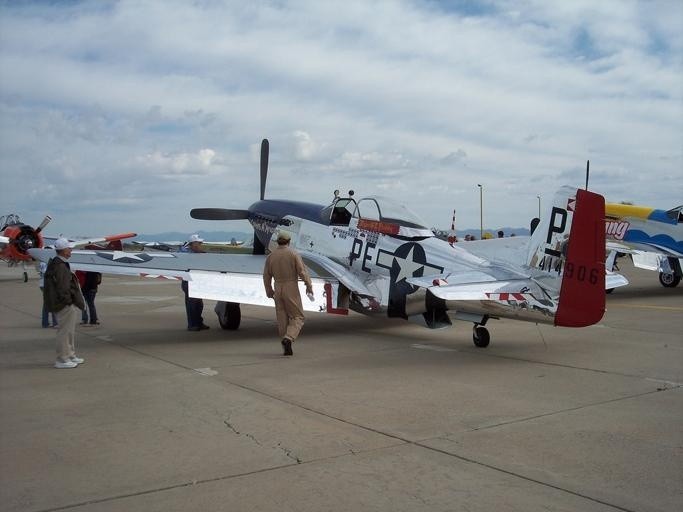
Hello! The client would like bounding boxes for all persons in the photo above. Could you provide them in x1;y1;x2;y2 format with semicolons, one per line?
74;268;101;325
261;230;315;356
42;237;85;369
447;231;515;245
37;246;57;329
179;235;208;333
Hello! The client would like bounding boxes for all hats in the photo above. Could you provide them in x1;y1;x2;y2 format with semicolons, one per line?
276;231;291;241
53;237;76;250
190;234;205;243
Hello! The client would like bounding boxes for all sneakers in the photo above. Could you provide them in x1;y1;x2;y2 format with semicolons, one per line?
281;336;294;356
53;357;84;369
186;321;210;332
78;319;102;328
